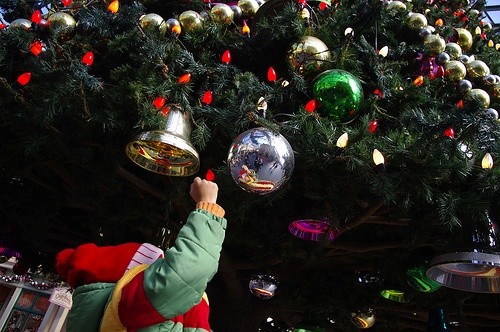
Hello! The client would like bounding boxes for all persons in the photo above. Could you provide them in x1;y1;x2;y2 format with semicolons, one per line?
55;177;228;332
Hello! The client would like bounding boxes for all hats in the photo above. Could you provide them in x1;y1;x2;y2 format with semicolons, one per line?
54;241;165;284
